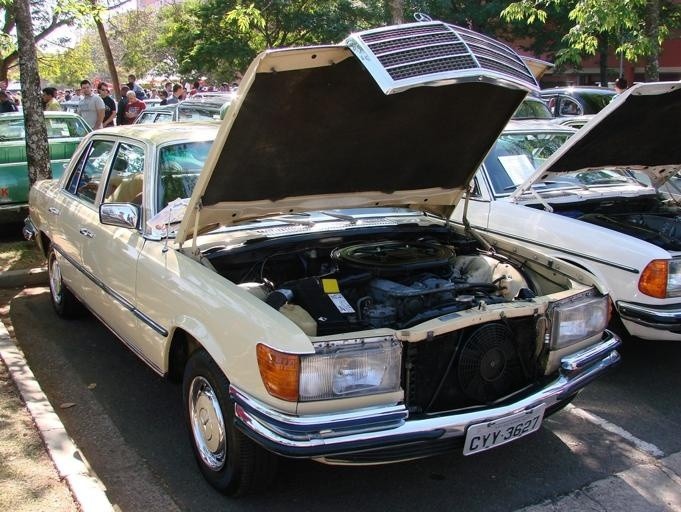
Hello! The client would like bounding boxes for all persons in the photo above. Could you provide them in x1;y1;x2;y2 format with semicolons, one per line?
611;78;628;101
0;74;239;132
